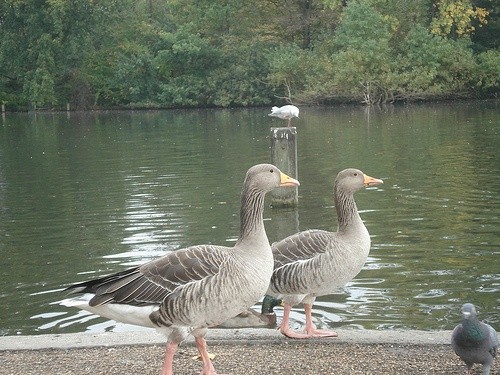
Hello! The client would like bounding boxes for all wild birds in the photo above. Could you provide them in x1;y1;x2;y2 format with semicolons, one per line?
267;104;300;128
266;169;384;339
48;163;301;375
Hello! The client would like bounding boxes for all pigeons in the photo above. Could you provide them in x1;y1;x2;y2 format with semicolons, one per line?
450;303;500;375
